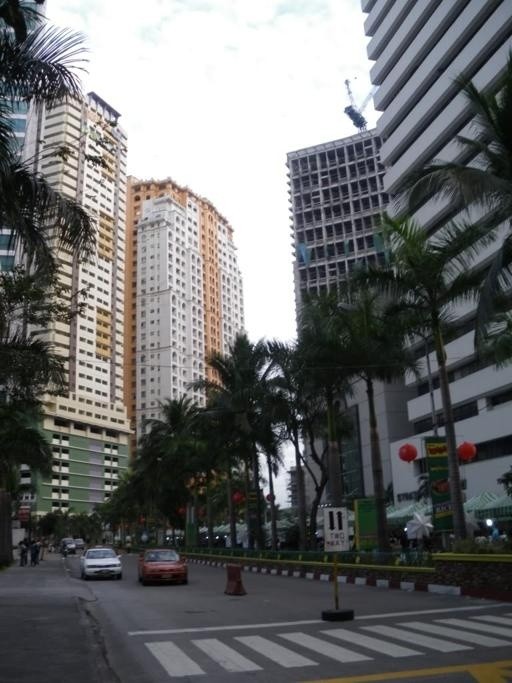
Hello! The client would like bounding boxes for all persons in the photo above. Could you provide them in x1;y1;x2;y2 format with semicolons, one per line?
18;537;41;568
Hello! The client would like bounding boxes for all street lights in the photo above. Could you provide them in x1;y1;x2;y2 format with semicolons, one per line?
335;301;449;552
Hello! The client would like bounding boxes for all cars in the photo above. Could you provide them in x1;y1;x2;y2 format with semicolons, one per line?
136;547;191;586
59;535;132;582
